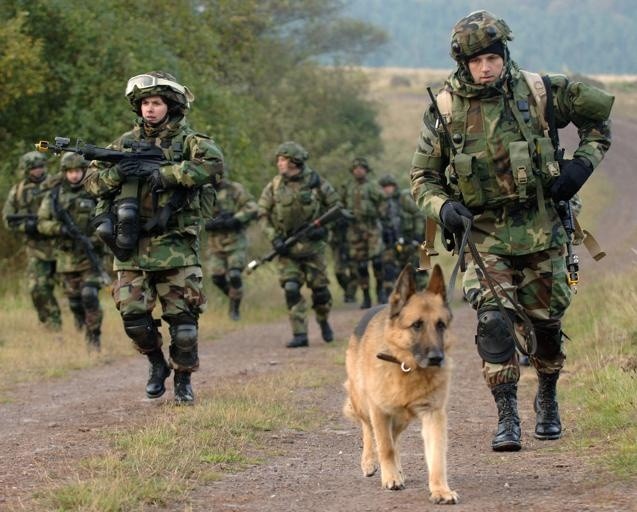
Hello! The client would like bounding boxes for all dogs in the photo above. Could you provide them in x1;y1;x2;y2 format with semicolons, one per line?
340;261;462;506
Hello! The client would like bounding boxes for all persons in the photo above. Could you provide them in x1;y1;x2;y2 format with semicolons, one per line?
82;71;224;407
338;157;387;309
257;141;344;348
330;227;360;302
2;151;67;333
35;150;110;358
204;180;258;321
377;174;427;293
409;10;616;453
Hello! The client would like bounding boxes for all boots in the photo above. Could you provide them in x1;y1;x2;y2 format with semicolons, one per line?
229;298;240;320
85;335;100;356
361;288;371;308
492;383;521;451
174;369;194;406
377;279;388;304
145;360;171;399
534;370;561;439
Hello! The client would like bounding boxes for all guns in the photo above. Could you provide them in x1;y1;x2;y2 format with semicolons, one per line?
56;208;111;286
35;137;170;193
532;148;578;294
245;205;356;275
7;214;37;226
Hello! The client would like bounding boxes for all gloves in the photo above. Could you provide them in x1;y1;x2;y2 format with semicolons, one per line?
441;202;473;233
308;228;327;241
61;225;73;239
118;158;139;177
146;169;163;194
550;157;593;204
272;238;288;256
225;218;235;227
28;222;38;235
214;217;225;228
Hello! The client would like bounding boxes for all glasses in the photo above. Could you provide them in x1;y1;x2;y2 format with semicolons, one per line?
126;75;194;102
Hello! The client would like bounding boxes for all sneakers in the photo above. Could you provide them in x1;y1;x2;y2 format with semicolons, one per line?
320;320;334;342
286;334;308;348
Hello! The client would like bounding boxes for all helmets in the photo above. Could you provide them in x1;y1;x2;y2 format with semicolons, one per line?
381;175;397;186
450;10;513;61
349;158;369;172
61;152;88;170
276;142;305;165
131;71;186;113
23;152;44;169
